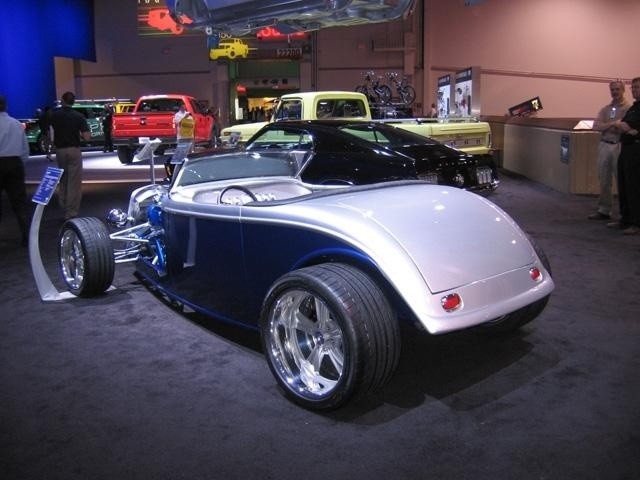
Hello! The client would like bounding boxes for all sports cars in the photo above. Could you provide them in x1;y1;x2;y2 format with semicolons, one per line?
58;116;557;410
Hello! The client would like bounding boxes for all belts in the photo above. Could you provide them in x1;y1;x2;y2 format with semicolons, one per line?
601;139;616;144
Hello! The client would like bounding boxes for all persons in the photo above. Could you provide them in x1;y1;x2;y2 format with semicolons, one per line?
430;103;437;118
243;106;273;123
606;77;640;236
39;106;55;162
43;91;93;223
173;101;194;155
35;108;42;119
588;76;632;220
100;103;115;152
0;94;34;249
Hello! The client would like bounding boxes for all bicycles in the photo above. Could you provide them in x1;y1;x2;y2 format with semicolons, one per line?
354;70;392;106
381;72;416;105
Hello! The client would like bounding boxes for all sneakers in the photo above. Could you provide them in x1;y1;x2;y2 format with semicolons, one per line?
589;212;640;235
